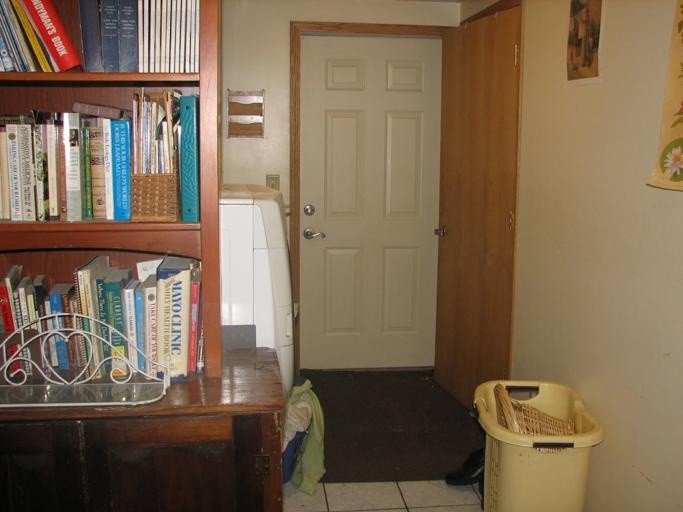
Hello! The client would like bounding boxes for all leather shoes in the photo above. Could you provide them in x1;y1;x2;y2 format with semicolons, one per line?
445;448;484;485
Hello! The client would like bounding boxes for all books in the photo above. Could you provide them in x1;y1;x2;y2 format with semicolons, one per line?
0;89;198;223
0;0;200;75
0;254;204;380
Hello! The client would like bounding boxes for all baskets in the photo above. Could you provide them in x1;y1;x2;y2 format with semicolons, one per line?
494;383;576;453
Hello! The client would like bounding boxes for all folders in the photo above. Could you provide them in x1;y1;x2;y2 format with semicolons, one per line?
180;96;200;224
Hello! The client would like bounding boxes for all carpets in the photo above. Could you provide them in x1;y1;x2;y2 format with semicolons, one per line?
295;369;481;483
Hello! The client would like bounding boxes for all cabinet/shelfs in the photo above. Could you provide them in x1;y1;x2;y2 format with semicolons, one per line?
0;346;282;512
0;1;223;381
